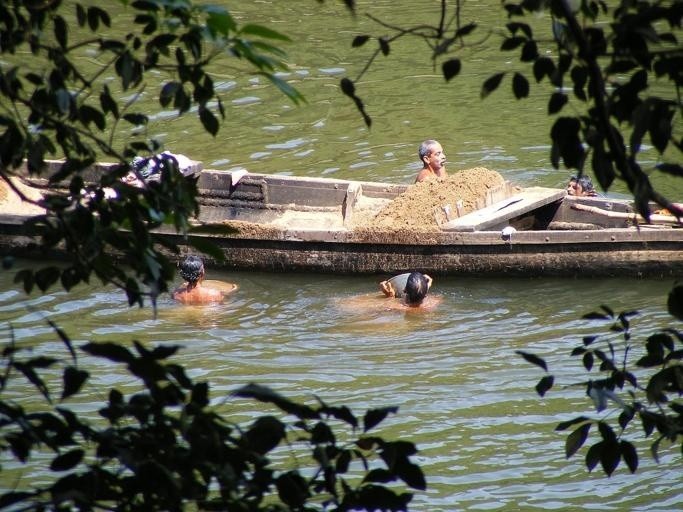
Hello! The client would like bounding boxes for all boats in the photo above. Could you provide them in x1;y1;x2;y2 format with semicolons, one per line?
1;156;682;279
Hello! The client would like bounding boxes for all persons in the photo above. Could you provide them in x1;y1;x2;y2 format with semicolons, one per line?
415;137;447;182
566;174;598;197
375;270;441;310
170;254;222;305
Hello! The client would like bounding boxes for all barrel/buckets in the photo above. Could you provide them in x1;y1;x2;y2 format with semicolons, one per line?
386;273;410;296
